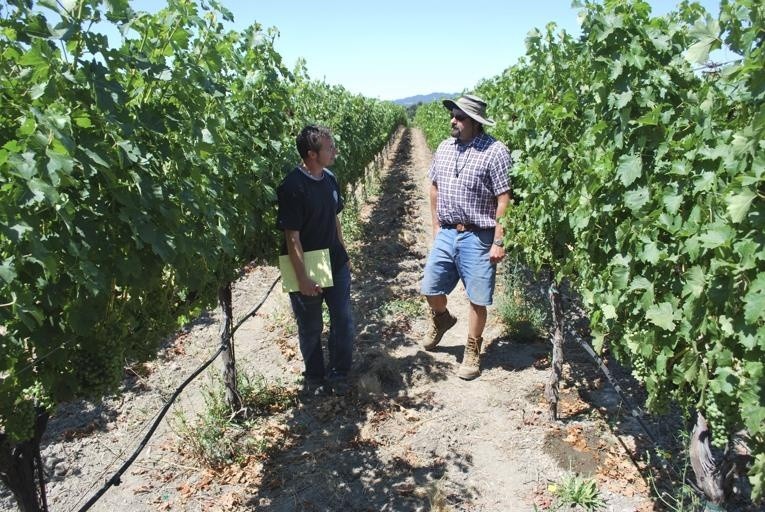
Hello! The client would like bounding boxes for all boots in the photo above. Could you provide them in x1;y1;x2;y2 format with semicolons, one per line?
455;334;483;380
423;310;458;351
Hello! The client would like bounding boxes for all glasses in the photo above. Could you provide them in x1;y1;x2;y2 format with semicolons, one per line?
450;112;470;122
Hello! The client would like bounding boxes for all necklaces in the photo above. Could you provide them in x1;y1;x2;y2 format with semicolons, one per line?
452;139;473;179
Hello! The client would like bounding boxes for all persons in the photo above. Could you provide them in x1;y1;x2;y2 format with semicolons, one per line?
276;124;377;399
419;93;512;382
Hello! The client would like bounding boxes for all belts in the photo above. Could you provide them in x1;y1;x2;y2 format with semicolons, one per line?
442;222;494;232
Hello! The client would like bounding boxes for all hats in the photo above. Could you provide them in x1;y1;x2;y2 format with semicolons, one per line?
442;94;497;128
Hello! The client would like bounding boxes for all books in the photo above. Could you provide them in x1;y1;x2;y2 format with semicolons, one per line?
276;248;335;294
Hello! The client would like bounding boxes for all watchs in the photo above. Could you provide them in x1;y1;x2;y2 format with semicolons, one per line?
492;239;505;247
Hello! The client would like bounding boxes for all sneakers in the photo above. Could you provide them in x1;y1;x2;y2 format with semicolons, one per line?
304;375;332;397
327;372;354;397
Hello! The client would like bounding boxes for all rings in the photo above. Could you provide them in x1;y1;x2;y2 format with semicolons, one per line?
310;293;314;296
